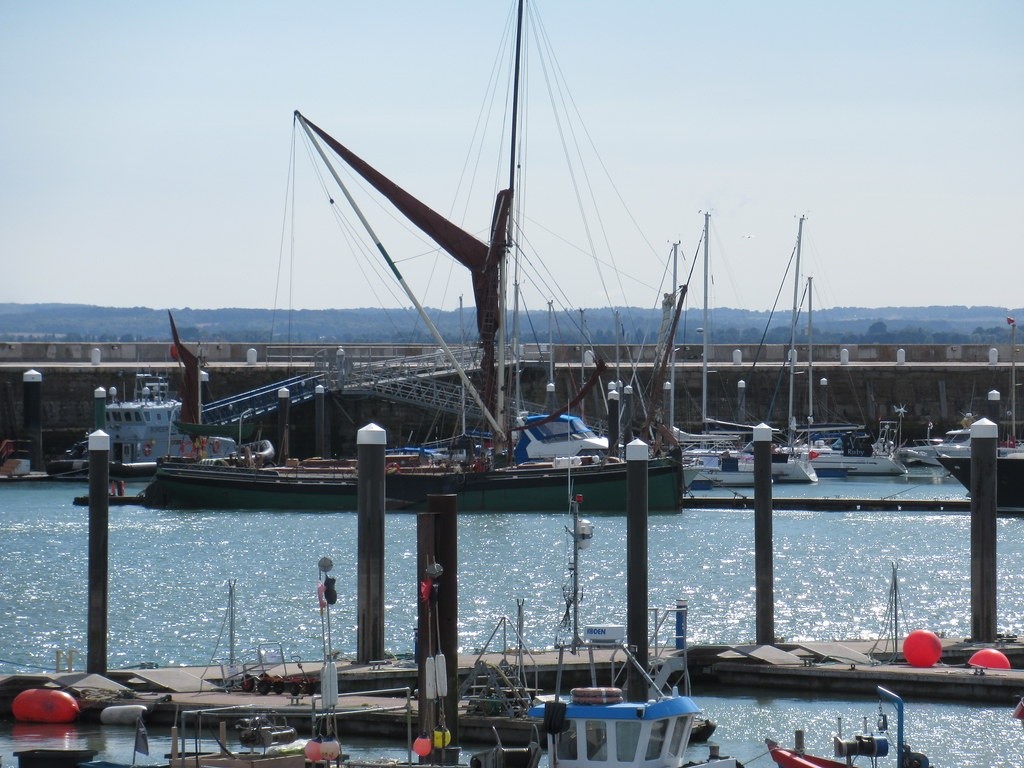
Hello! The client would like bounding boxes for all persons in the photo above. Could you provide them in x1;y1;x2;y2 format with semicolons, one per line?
960;413;976;428
719;449;730;457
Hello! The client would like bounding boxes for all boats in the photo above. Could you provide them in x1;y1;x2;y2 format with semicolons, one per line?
525;644;737;768
171;720;306;768
765;684;929;768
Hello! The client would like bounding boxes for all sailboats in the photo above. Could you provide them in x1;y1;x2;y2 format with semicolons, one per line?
50;1;1024;514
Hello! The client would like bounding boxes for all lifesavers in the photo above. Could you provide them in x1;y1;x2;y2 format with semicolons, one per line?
387;460;400;475
455;473;466;485
212;439;221;453
142;442;153;457
179;440;197;456
571;687;624;706
